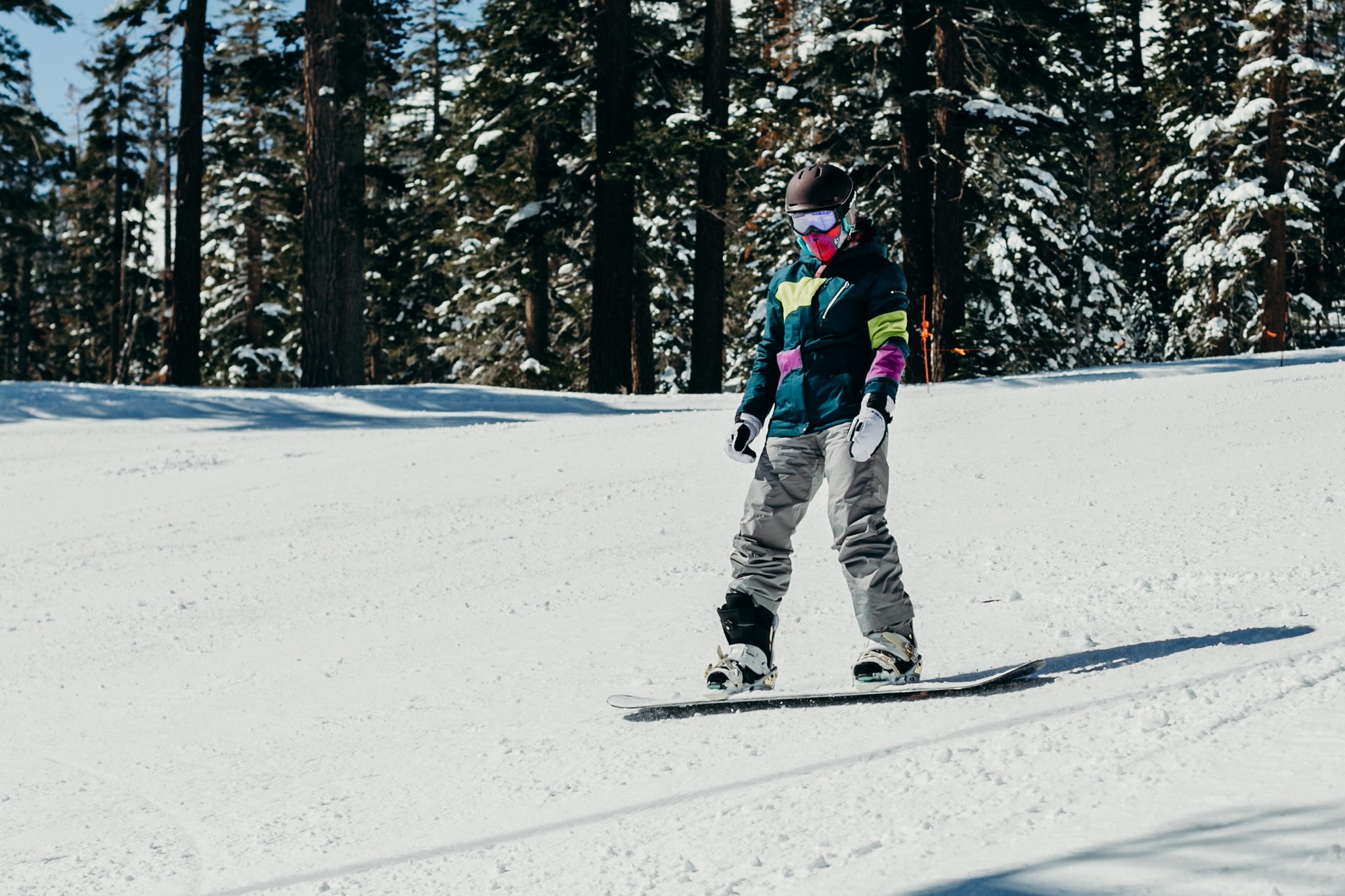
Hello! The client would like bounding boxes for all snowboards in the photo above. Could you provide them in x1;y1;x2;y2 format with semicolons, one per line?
604;654;1047;710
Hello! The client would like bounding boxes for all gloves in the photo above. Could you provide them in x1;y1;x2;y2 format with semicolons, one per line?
847;392;895;462
724;411;761;464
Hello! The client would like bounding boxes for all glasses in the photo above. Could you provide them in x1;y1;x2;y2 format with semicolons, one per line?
786;203;842;236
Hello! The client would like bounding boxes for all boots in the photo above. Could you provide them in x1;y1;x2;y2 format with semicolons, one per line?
853;616;917;681
708;591;772;690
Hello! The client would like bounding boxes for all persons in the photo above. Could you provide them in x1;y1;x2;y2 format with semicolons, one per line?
701;163;922;702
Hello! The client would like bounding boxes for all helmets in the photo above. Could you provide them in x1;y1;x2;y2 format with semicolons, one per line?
784;163;854;213
